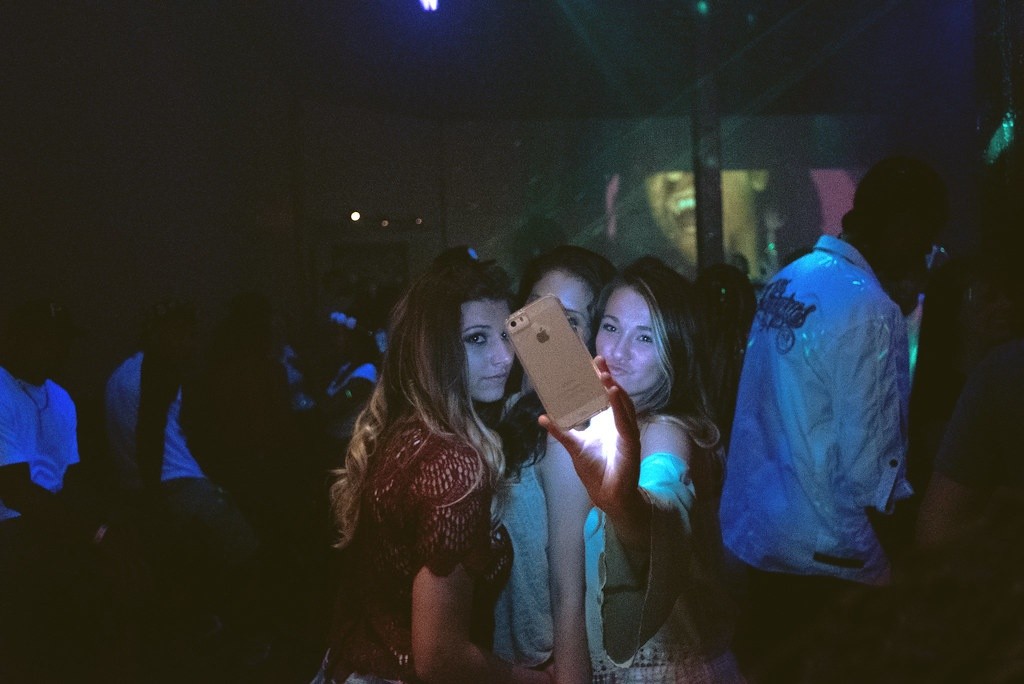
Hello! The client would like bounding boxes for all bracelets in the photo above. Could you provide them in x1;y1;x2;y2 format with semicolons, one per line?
92;522;110;546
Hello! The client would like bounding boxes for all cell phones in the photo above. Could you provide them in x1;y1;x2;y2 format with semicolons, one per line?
503;294;610;431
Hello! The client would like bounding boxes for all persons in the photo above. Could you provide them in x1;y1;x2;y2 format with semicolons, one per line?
536;255;746;684
310;245;553;684
180;314;347;636
0;300;132;586
103;290;259;554
279;304;378;439
916;336;1024;682
483;245;620;683
690;263;754;456
605;166;826;284
965;172;1024;378
718;154;939;684
